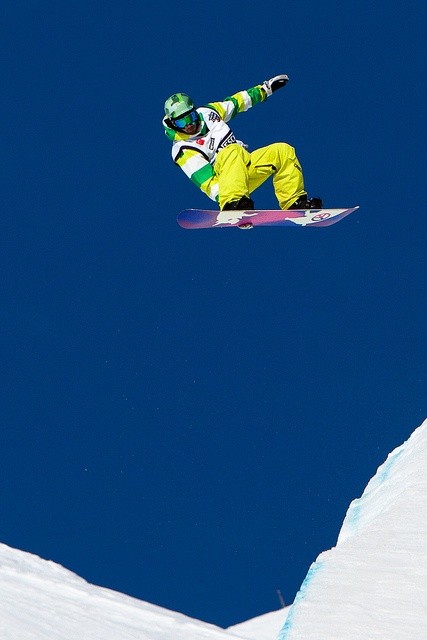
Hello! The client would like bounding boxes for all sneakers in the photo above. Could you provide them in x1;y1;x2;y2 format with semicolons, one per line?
222;195;254;208
289;195;323;208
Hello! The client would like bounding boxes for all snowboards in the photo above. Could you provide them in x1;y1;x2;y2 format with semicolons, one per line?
177;206;360;230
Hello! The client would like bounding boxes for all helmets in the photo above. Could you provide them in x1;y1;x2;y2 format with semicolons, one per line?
164;93;194;119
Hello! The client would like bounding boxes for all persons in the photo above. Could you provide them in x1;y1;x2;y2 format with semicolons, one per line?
162;74;323;230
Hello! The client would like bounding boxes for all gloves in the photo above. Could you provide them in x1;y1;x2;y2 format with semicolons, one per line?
262;74;289;97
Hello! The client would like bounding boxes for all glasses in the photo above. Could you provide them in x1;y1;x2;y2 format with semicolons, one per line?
173;111;198;129
